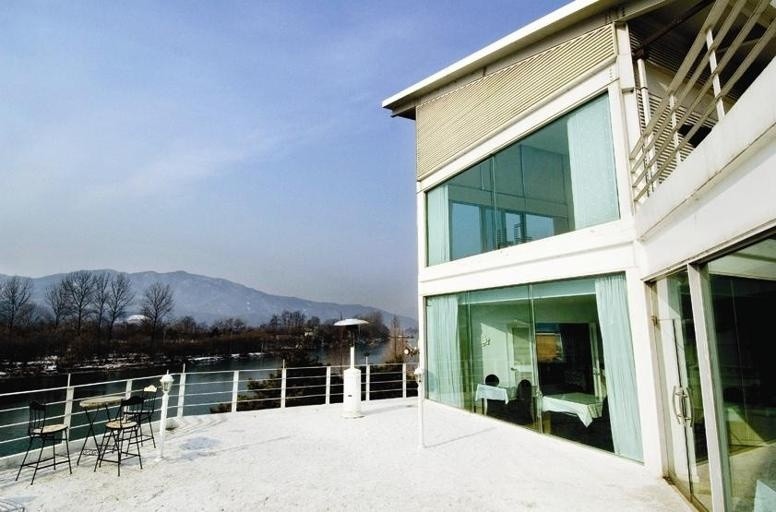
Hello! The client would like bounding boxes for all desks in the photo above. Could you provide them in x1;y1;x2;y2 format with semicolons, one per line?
478;384;538;422
543;392;604;434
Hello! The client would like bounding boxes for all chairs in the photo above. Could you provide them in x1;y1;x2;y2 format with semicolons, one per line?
589;396;612;440
16;384;157;485
485;374;533;425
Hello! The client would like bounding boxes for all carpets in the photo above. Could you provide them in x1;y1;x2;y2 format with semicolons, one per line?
690;439;776;511
